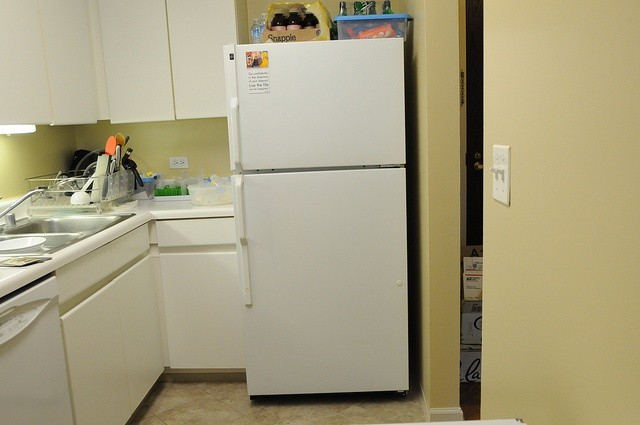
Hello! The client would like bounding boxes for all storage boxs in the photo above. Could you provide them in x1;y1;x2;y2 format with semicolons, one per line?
334;13;413;42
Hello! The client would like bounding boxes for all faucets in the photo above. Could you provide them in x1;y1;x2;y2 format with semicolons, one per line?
1;186;49;230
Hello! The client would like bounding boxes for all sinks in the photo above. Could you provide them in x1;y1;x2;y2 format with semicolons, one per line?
1;235;78;251
11;210;119;233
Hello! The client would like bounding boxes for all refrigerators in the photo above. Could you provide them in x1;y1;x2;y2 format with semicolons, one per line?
222;39;411;401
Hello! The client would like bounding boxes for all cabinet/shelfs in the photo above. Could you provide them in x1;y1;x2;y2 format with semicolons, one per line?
0;0;98;126
97;0;238;124
55;224;166;424
151;217;247;375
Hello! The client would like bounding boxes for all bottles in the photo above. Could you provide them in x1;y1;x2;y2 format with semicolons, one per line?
312;15;318;27
382;2;392;14
337;2;347;15
250;19;261;44
366;1;375;15
270;8;286;32
287;7;301;30
353;2;363;15
302;10;314;28
258;12;267;41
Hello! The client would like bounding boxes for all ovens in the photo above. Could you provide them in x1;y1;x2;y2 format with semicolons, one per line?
0;270;76;425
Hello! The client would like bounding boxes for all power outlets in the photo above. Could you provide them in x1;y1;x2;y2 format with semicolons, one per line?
169;157;188;168
490;144;511;205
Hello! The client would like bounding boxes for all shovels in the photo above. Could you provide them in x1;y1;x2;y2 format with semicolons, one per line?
105;136;116;156
115;132;125;152
